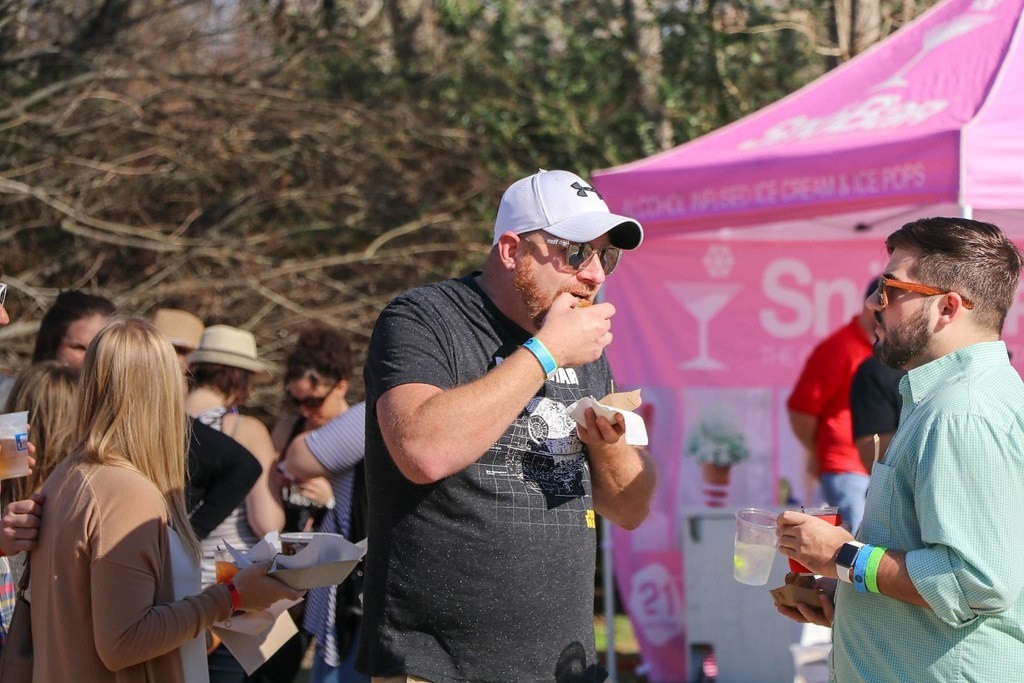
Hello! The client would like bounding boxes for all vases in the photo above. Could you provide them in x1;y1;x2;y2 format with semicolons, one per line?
699;462;731;508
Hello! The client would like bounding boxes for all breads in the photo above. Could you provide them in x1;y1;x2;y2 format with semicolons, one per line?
785;571;817;590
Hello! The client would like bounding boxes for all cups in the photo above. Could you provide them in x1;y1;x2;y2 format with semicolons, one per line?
0;410;30;480
212;548;250;611
731;507;781;587
789;506;841;577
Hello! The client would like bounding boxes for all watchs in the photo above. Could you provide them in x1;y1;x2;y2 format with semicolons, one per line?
833;540;866;584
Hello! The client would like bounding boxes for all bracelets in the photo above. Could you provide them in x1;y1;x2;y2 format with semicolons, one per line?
864;547;890;596
223;579;241;612
522;337;558;381
853;544;874;593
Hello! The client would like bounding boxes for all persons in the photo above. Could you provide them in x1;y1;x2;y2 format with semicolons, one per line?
777;217;1024;683
0;282;365;683
782;276;907;537
354;170;656;683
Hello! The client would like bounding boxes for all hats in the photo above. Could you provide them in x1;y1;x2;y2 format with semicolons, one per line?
153;309;204;350
187;324;273;385
492;170;645;251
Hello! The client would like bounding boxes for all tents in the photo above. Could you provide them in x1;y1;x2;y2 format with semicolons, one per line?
589;0;1024;683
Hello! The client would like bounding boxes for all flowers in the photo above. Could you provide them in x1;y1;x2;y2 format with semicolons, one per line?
685;421;747;466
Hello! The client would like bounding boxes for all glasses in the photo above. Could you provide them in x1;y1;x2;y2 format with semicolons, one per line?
0;283;8;306
877;276;974;311
290;380;339;410
521;235;623;276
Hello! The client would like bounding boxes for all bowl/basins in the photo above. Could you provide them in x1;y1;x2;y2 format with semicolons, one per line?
279;532;344;556
